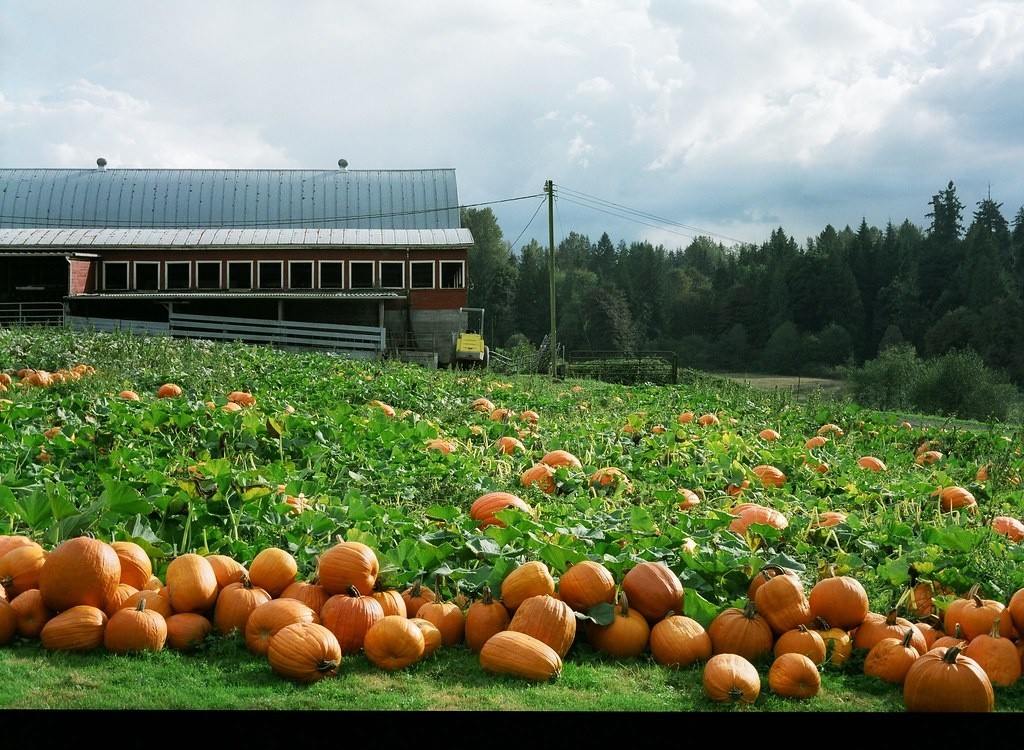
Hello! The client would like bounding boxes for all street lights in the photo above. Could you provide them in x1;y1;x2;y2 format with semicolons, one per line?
543;179;557;377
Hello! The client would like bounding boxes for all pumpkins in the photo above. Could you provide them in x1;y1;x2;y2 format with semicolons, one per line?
0;366;1024;711
